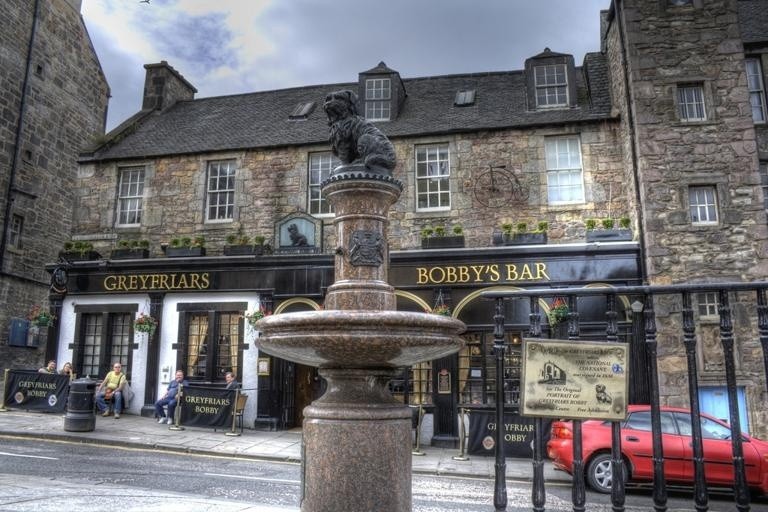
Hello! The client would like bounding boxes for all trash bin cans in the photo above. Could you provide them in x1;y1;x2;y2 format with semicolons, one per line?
64;378;97;432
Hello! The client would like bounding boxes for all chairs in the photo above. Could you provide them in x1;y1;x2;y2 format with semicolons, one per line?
231;395;249;432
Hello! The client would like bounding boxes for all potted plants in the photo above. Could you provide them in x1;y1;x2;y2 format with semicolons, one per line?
421;216;634;250
60;233;269;263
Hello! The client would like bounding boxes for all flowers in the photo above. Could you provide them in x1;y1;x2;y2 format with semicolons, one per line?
128;313;159;336
424;304;451;316
548;298;568;329
27;304;57;329
249;303;273;325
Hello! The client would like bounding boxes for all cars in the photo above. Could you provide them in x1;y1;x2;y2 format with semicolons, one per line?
546;404;768;503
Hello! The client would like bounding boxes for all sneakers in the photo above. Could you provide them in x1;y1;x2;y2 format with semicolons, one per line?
158;417;166;424
101;410;110;416
113;410;120;419
167;417;172;425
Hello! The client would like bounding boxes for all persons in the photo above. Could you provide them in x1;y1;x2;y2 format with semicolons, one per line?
60;362;73;387
154;369;190;424
224;371;242;394
37;360;60;375
96;362;128;419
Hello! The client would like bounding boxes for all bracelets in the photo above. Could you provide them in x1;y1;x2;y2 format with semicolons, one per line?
112;391;115;395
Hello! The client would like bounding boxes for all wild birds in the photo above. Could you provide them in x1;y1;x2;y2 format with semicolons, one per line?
140;0;150;5
